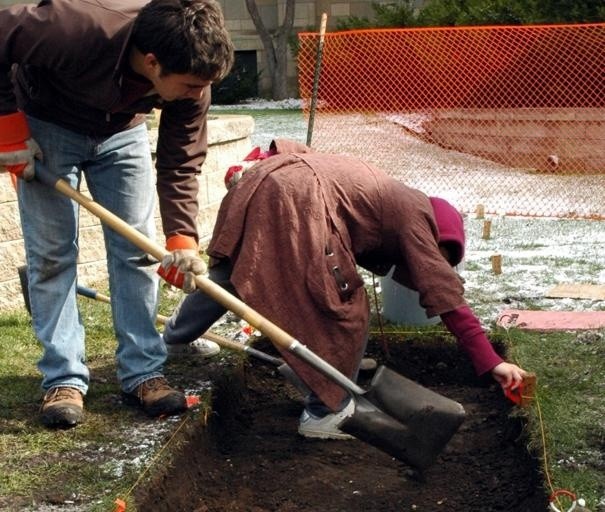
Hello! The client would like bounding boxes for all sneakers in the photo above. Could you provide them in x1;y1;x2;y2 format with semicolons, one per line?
297;397;358;440
131;377;187;414
38;387;84;429
159;334;220;357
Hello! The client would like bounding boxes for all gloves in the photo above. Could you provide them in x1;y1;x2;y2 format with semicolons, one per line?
0;112;43;181
224;146;261;191
156;235;207;294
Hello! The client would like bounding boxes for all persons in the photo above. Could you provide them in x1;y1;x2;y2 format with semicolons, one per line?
164;139;528;439
0;0;235;427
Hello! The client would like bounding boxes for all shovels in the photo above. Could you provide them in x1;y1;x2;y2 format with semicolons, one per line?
34;158;465;475
18;263;314;404
